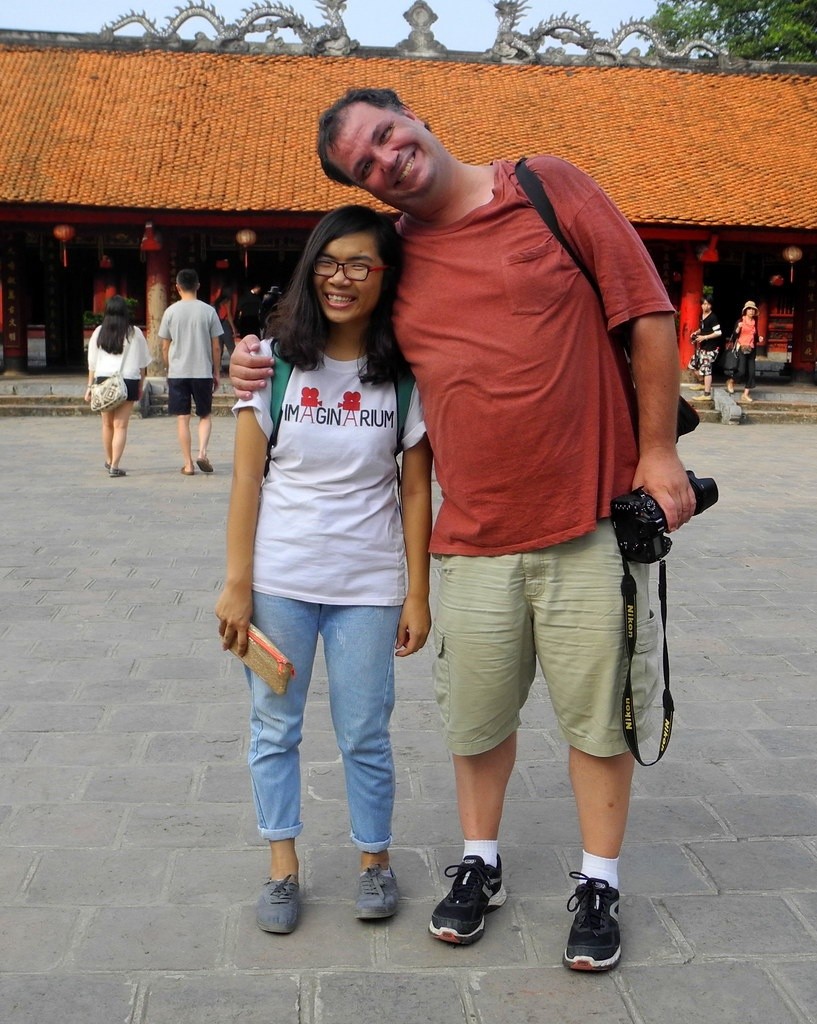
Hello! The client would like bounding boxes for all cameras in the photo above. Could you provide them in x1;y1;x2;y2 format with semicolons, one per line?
611;469;719;564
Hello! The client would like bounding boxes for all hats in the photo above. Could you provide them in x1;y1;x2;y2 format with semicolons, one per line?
742;301;760;316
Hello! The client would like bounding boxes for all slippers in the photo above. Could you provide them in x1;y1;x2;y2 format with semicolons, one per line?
181;465;195;474
197;459;213;472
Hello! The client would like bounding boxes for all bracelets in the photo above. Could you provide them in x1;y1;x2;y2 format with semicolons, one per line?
88;385;94;388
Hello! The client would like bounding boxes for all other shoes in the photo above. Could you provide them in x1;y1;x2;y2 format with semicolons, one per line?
109;467;126;476
692;395;712;400
726;379;734;394
690;384;705;391
104;461;111;469
741;394;753;402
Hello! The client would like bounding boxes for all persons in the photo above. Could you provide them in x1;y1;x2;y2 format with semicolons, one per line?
214;203;434;935
688;293;723;404
725;301;764;406
211;260;237;374
228;82;698;976
158;270;225;475
84;296;152;477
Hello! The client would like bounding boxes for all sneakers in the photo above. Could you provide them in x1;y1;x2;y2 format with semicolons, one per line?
354;866;400;918
257;876;300;933
562;883;622;971
428;853;507;946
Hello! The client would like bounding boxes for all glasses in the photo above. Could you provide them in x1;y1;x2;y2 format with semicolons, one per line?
312;259;388;281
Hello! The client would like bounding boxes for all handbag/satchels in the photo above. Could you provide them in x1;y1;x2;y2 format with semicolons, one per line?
227;623;296;695
675;394;698;444
89;372;127;412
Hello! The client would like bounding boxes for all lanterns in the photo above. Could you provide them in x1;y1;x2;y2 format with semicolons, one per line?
780;245;803;283
234;227;258;268
53;225;76;267
